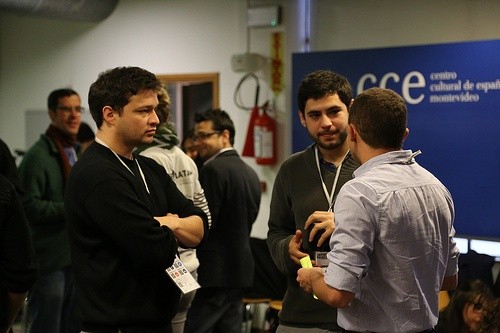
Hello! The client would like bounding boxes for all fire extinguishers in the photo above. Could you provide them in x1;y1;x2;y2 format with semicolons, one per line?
234;72;279;165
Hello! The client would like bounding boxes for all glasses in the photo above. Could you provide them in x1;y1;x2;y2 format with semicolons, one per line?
191;131;221;144
56;106;85;117
470;302;491;316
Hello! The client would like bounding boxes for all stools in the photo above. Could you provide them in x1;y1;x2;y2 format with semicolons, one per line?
243;299;283;333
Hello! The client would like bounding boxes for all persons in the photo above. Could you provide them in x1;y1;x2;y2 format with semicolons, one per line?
267;70;361;333
16;88;79;333
182;108;262;333
0;174;40;333
449;278;500;333
132;86;213;333
297;87;459;333
62;67;209;333
